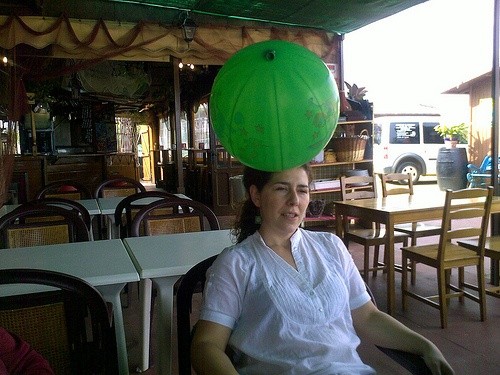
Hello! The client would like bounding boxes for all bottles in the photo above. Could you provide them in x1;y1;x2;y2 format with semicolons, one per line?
338;92;352;116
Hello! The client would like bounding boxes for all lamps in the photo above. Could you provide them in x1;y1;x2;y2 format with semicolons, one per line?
178;11;198;48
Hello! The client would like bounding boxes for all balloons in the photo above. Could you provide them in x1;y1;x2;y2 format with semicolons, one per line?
209;40;340;172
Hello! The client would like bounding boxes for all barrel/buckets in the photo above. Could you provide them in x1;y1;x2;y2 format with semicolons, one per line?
436;147;468;191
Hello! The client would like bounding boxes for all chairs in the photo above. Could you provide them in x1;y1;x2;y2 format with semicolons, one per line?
0;171;500;375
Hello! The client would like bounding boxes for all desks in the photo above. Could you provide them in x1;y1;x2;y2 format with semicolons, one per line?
334;192;500;314
0;236;140;375
125;229;241;371
0;199;101;242
99;192;193;239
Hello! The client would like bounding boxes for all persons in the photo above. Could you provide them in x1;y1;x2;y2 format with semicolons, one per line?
190;164;456;375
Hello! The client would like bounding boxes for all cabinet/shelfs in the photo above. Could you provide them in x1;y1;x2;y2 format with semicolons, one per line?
296;119;377;228
16;153;140;188
58;106;118;152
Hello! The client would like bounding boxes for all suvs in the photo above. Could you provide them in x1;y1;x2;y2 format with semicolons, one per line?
373;115;472;186
